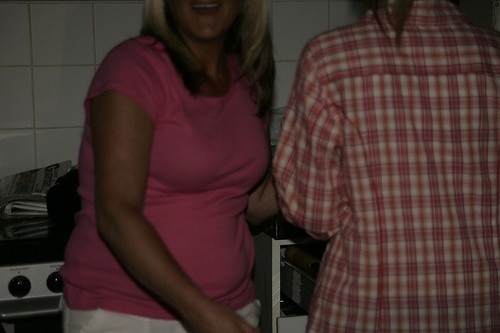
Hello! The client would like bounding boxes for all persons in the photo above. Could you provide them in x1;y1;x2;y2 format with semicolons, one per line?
272;0;500;333
61;0;280;333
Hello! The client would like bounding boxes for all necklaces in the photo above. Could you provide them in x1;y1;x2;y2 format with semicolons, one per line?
187;62;228;88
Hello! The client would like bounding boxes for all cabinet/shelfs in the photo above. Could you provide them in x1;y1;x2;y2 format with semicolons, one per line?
253;228;329;333
